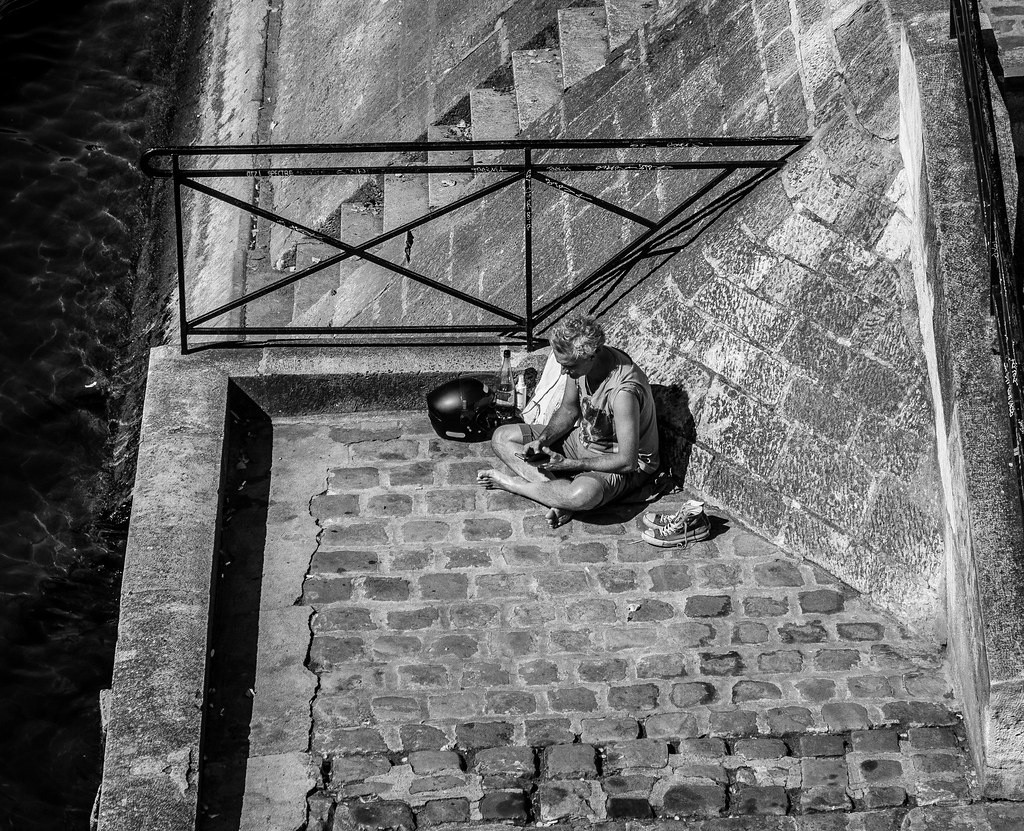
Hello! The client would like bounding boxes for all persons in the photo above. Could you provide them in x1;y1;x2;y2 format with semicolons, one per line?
476;315;659;529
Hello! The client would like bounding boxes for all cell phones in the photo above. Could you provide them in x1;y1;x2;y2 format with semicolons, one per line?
515;453;538;461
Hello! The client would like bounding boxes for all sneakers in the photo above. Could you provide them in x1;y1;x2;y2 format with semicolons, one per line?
642;500;712;550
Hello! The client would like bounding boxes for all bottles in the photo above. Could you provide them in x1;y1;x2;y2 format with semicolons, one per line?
494;350;515;420
516;375;526;410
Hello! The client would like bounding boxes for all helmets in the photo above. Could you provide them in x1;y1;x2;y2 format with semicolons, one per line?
426;377;497;443
